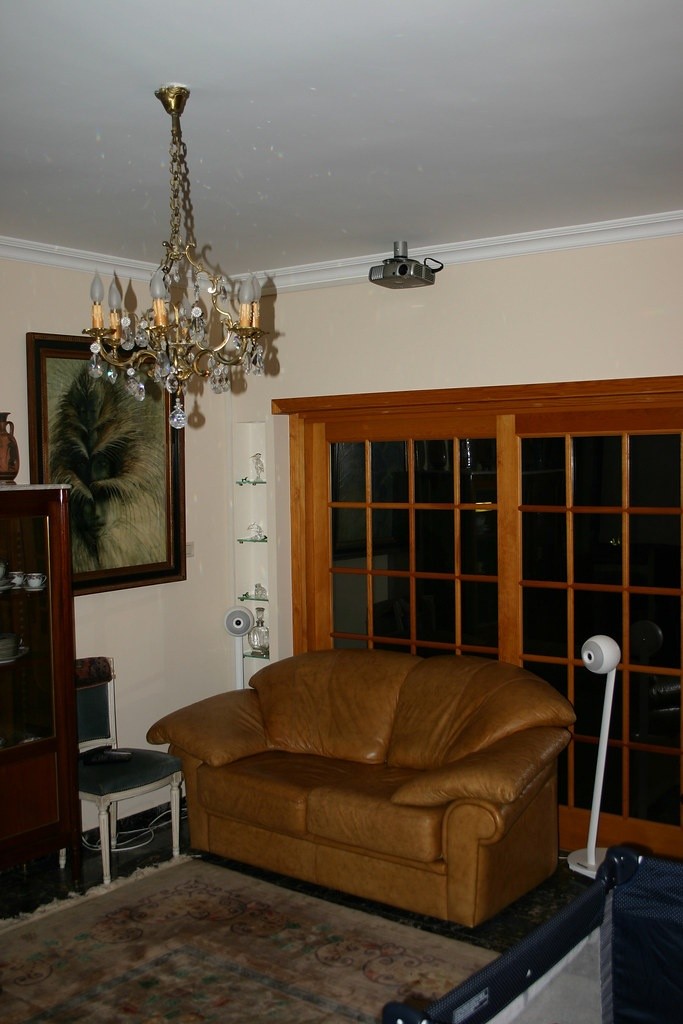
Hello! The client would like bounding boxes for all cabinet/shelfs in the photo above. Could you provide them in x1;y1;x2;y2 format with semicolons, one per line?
237;480;269;659
0;483;85;870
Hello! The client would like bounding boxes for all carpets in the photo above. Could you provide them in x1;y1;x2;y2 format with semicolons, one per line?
0;856;500;1024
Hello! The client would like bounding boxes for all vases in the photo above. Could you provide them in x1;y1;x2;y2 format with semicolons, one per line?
0;412;19;486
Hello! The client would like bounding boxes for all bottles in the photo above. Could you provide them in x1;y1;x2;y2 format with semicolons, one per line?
248;608;269;651
0;412;19;485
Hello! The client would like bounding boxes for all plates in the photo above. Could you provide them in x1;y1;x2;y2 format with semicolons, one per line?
23;587;44;591
0;647;29;664
0;582;16;590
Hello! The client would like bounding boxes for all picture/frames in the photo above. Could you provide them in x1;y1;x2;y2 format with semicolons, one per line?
26;331;187;598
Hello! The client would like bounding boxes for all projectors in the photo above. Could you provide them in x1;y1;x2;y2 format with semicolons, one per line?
369;261;435;289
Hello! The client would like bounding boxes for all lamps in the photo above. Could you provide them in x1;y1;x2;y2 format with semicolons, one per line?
80;82;270;430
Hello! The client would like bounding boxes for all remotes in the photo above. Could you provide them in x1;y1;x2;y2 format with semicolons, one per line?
82;749;133;766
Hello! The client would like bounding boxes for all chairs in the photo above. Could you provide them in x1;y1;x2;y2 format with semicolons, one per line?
74;656;184;883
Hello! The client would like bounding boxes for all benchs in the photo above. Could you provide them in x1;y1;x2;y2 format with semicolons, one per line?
146;650;577;929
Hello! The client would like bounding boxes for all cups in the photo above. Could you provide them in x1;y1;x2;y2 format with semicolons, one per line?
9;571;27;586
0;562;6;580
26;573;47;587
